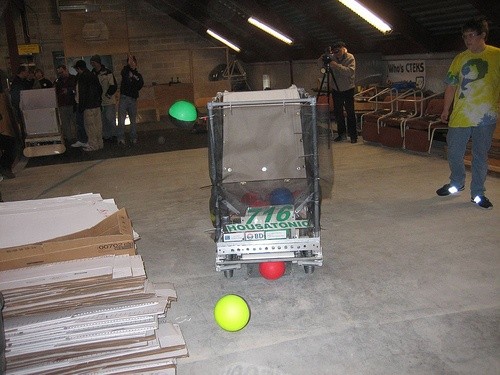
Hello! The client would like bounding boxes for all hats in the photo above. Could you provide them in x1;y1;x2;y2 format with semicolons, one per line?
333;41;347;48
91;55;101;64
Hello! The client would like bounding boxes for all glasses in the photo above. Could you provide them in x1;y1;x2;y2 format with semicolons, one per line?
461;32;480;40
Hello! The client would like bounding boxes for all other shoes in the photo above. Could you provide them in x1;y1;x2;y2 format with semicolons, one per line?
82;145;96;152
471;195;493;210
436;183;465;197
334;136;347;142
70;141;88;148
350;136;358;143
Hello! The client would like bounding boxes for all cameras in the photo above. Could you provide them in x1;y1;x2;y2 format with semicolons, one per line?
321;54;334;62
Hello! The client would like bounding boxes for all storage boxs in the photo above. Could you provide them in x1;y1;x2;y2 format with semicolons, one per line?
20;87;62;135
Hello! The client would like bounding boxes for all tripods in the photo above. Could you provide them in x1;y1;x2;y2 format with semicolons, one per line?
316;61;346;149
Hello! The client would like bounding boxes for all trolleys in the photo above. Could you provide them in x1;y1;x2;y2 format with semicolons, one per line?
207;84;324;278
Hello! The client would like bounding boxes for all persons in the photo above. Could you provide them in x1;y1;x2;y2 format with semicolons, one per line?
436;18;500;209
10;65;53;126
318;41;358;143
55;60;104;151
117;56;144;145
89;55;118;143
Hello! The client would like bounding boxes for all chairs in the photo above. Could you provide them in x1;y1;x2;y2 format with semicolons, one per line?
353;87;454;156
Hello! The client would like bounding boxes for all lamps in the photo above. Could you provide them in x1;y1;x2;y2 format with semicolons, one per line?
337;0;395;35
247;16;295;46
206;28;241;53
